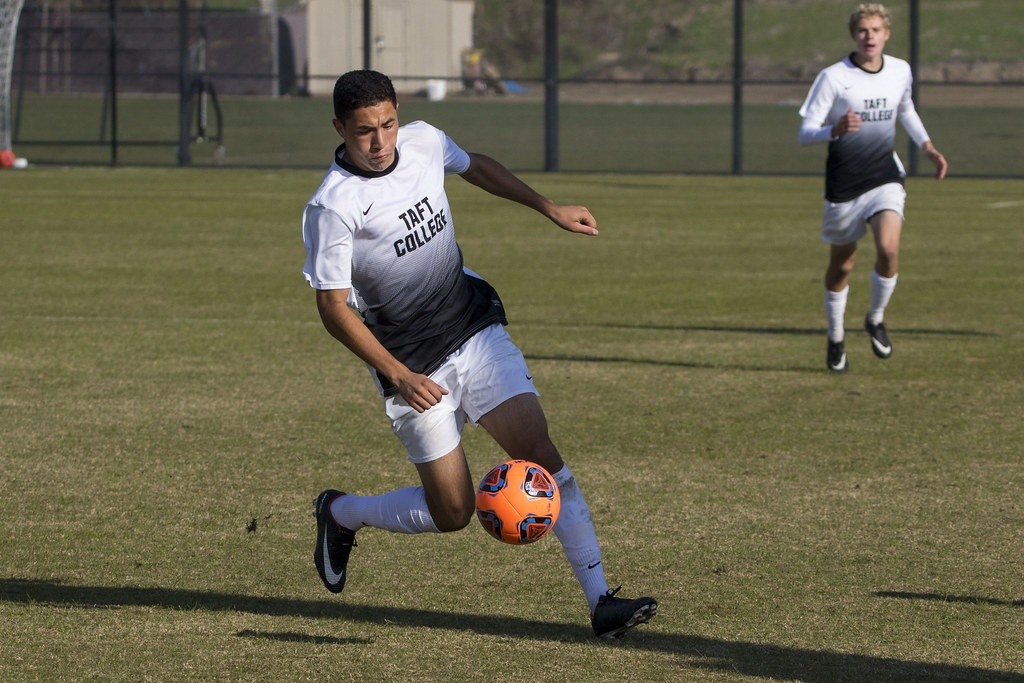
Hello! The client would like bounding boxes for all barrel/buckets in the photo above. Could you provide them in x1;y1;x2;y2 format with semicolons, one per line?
425;79;446;100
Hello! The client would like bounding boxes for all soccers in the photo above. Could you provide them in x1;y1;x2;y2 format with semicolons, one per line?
473;458;561;547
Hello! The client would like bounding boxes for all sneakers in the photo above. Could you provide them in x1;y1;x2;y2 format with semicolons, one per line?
864;313;893;359
313;489;358;594
826;327;849;372
592;584;658;639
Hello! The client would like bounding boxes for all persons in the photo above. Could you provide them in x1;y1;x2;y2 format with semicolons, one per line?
302;70;658;641
799;0;948;372
